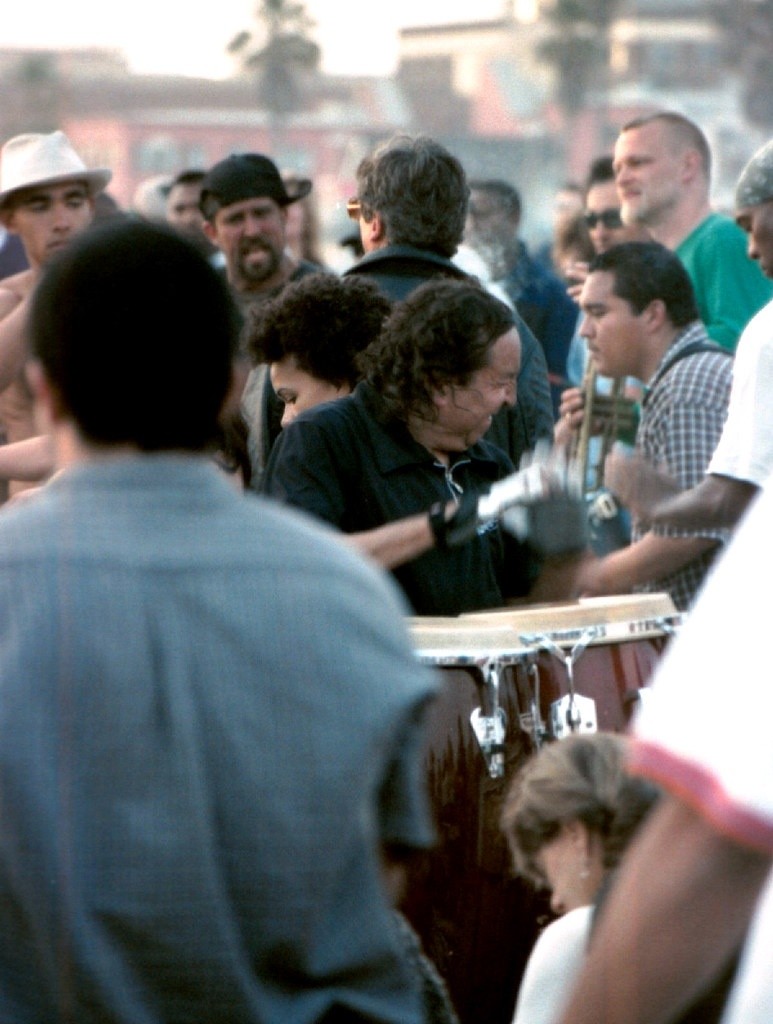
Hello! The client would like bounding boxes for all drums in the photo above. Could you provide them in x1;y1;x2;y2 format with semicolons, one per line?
404;615;538;834
458;593;685;735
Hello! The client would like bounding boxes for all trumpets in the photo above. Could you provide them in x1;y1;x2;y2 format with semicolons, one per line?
560;356;636;507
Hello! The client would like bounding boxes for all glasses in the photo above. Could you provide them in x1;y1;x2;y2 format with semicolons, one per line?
347;196;363;218
583;208;621;229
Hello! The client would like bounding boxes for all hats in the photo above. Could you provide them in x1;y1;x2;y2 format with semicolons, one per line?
0;131;113;205
198;154;311;223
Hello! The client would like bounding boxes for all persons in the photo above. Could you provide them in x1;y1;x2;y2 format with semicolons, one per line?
599;136;772;532
262;274;554;618
0;128;562;499
0;216;444;1024
502;729;658;1024
562;463;773;1023
530;112;772;650
